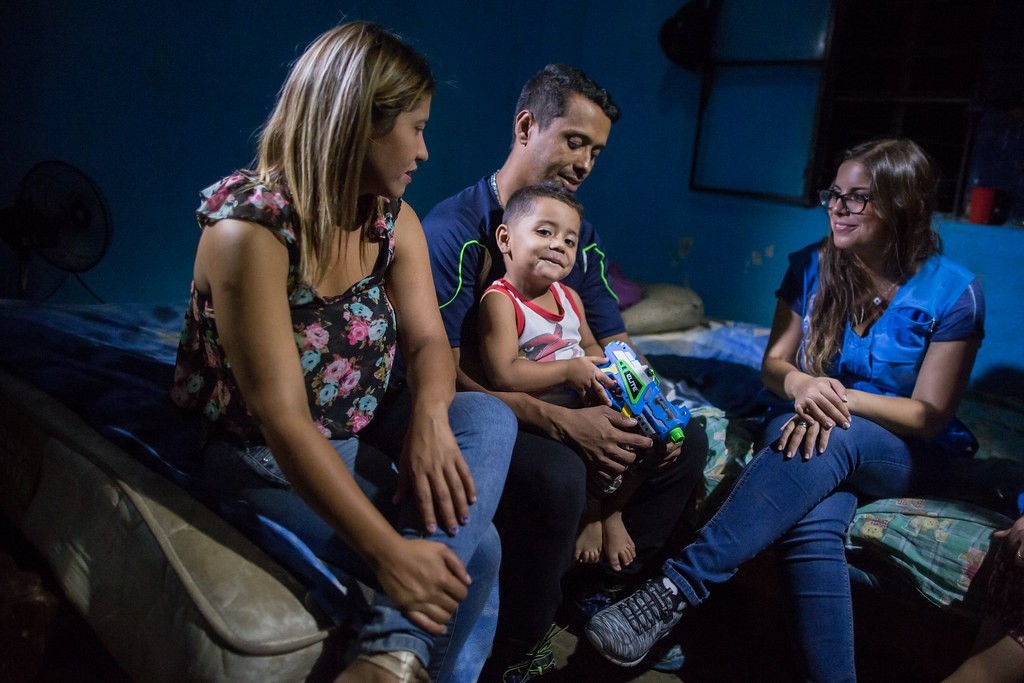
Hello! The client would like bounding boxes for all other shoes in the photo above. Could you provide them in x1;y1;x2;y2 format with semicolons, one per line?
331;650;432;683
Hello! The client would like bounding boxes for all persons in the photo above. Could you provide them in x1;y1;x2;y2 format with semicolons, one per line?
477;185;648;571
587;137;986;683
422;64;708;683
157;23;518;683
941;518;1024;683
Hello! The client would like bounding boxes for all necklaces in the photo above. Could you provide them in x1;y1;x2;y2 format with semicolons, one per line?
491;170;501;205
866;280;899;306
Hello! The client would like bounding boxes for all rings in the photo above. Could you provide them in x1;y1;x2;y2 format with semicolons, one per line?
1018;549;1023;559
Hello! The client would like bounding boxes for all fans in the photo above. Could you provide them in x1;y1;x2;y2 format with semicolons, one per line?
0;161;112;301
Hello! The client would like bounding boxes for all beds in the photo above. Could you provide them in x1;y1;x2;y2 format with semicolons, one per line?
1;298;1024;683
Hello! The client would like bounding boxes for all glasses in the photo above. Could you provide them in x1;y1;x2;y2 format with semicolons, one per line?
817;188;874;214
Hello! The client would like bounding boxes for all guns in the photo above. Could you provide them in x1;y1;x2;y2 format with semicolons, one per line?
595;339;693;447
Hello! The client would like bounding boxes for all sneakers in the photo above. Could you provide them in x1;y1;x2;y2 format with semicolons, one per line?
485;623;569;683
583;575;688;667
565;592;685;670
